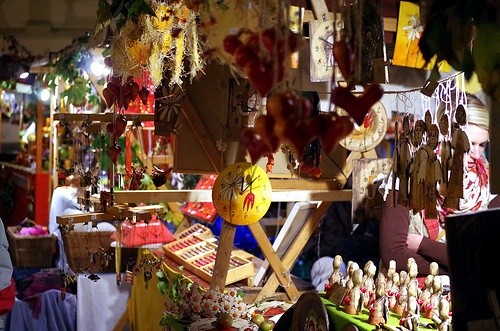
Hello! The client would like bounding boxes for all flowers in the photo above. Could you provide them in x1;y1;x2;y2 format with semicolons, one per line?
402;16;424;41
139;251;278;331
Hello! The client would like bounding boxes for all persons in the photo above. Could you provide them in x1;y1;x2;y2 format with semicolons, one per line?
0;218;15;331
379;92;500;278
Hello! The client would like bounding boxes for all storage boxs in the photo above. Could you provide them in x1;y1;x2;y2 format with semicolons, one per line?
162;223;255;286
59;225;115;272
172;57;244;174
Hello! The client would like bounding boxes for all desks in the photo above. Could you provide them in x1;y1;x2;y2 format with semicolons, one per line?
130;238;318;331
76;272;131;331
319;291;452;331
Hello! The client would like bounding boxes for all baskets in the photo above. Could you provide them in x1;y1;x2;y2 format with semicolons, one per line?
6;217;56;268
61;227;116;273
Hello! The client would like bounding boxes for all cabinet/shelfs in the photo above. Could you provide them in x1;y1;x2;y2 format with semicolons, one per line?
0;103;53;230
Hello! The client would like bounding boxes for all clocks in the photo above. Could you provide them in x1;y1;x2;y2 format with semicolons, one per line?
212;162;272;225
333;93;388;152
352;158;395;223
309;20;389;84
228;78;262;128
